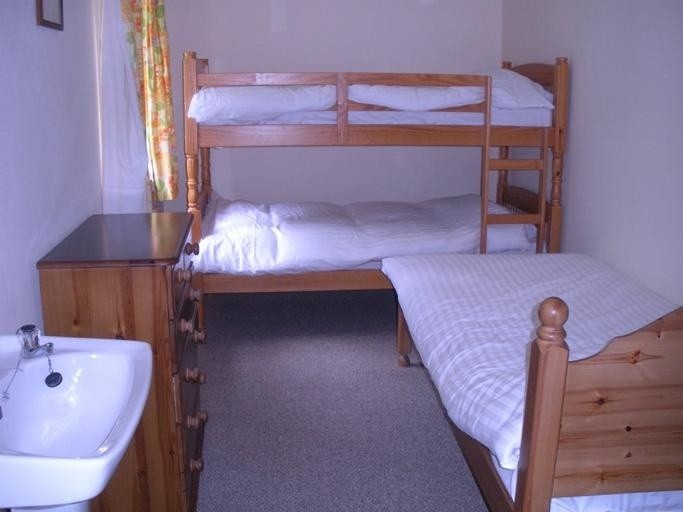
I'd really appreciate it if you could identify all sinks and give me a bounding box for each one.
[0,335,154,510]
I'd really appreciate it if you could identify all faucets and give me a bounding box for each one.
[17,324,54,359]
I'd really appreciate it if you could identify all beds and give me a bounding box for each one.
[181,52,569,331]
[398,252,682,512]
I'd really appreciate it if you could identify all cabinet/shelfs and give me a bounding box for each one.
[35,212,210,512]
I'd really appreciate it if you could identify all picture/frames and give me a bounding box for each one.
[36,0,63,30]
